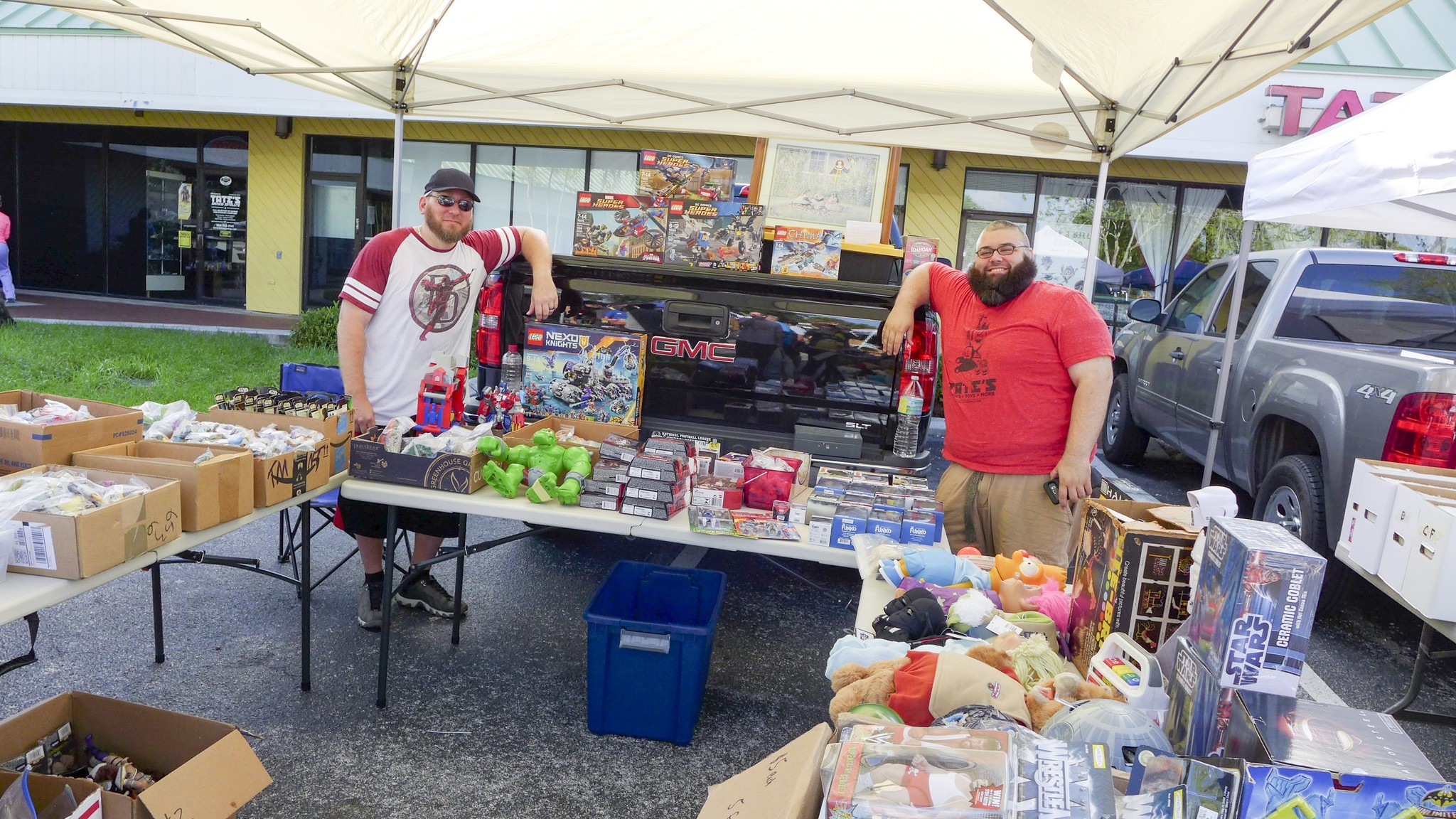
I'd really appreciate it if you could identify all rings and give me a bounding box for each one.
[549,308,555,310]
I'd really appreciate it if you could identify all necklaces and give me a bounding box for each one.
[418,225,425,238]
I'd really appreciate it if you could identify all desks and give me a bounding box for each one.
[1333,542,1456,730]
[0,468,353,692]
[341,477,955,716]
[849,567,1073,648]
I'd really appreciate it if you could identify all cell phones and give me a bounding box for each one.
[1043,477,1070,505]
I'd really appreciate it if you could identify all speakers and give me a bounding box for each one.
[276,116,293,138]
[933,150,947,169]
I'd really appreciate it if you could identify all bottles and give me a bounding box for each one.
[893,376,925,458]
[500,344,522,393]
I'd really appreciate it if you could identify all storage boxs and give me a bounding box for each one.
[0,319,1456,819]
[571,148,844,281]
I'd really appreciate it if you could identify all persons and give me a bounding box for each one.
[882,220,1117,569]
[334,168,559,629]
[0,196,17,304]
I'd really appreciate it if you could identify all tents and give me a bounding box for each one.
[1026,225,1209,304]
[1198,68,1456,492]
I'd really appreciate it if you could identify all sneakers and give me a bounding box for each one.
[396,564,468,618]
[357,581,391,629]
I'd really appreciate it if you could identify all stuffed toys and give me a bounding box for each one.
[822,547,1127,739]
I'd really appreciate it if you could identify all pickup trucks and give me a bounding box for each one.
[1102,245,1455,620]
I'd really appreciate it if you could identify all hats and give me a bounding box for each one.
[424,168,481,204]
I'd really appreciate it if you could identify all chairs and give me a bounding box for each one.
[279,361,414,598]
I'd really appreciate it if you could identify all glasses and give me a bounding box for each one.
[427,193,474,211]
[976,245,1029,259]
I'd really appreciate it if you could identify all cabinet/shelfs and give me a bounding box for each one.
[146,170,186,297]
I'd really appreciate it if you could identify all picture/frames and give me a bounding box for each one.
[746,138,903,244]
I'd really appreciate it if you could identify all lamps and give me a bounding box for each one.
[930,150,947,172]
[274,116,293,139]
